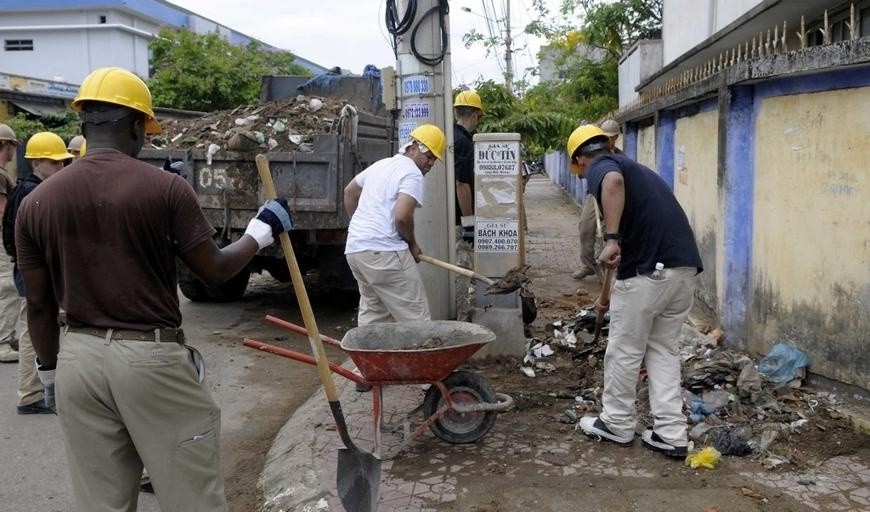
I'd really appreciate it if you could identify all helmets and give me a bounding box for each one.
[66,135,88,158]
[598,119,622,138]
[23,129,78,162]
[407,123,449,165]
[67,65,168,140]
[451,89,485,115]
[564,123,612,174]
[0,123,22,148]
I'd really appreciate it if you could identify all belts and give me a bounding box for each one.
[58,322,189,347]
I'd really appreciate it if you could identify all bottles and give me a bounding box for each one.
[649,262,666,281]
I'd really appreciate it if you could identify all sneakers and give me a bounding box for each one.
[0,340,20,366]
[419,383,434,393]
[352,379,376,393]
[577,415,637,446]
[570,265,596,280]
[640,429,691,460]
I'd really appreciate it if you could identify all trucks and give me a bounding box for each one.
[134,75,398,309]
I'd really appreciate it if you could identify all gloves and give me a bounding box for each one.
[241,195,298,253]
[36,365,59,417]
[459,213,477,248]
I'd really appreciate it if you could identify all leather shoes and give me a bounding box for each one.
[13,395,61,416]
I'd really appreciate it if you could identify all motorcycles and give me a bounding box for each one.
[522,153,546,176]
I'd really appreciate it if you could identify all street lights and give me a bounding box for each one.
[459,1,512,133]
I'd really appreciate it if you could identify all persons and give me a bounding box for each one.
[453,89,486,321]
[0,124,88,413]
[343,125,432,392]
[566,124,703,459]
[140,467,155,494]
[571,118,628,279]
[13,68,293,512]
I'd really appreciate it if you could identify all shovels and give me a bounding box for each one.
[417,254,529,296]
[255,153,382,512]
[572,253,619,359]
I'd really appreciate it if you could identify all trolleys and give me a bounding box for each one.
[243,315,516,460]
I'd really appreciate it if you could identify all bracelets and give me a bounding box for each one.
[603,234,619,242]
